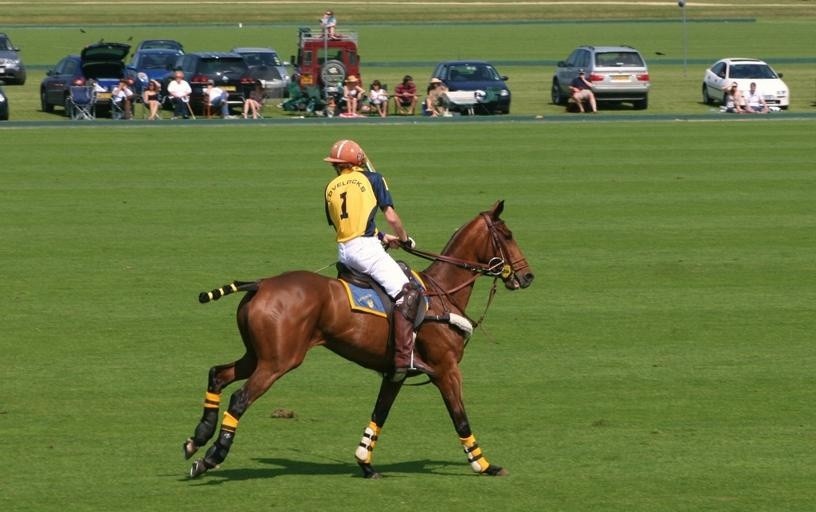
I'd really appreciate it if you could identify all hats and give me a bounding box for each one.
[344,75,357,82]
[429,77,441,83]
[578,67,585,74]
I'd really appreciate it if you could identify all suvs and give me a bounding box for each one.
[548,43,652,108]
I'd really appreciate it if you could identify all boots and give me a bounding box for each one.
[391,304,437,375]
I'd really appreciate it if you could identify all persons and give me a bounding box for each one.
[113,78,134,119]
[322,137,438,374]
[144,79,162,118]
[204,80,230,118]
[318,7,340,40]
[743,81,772,116]
[370,80,389,118]
[168,71,192,119]
[722,79,744,115]
[241,79,261,118]
[568,68,600,114]
[394,75,417,114]
[427,78,450,116]
[342,75,365,115]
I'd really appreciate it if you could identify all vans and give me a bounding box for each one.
[294,29,363,95]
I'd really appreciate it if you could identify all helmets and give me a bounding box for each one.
[322,139,366,165]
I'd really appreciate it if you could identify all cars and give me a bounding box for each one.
[38,38,289,125]
[432,61,513,116]
[0,33,28,84]
[1,90,13,120]
[701,53,790,107]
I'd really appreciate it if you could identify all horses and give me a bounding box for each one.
[183,199,534,480]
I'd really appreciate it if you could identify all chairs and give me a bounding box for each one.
[473,86,501,115]
[202,87,230,119]
[67,84,95,121]
[335,83,389,115]
[109,83,167,120]
